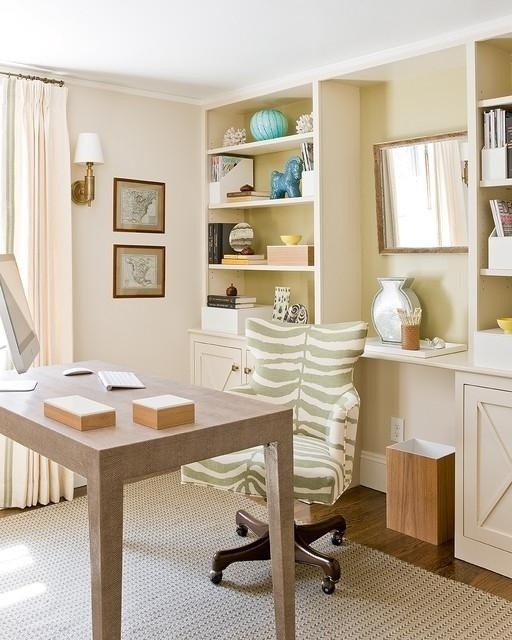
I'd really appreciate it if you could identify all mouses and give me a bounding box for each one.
[63,368,92,376]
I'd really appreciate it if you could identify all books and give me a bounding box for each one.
[221,258,266,265]
[209,294,257,304]
[226,191,270,197]
[483,109,512,237]
[225,196,269,202]
[207,300,256,309]
[211,155,246,182]
[223,253,265,259]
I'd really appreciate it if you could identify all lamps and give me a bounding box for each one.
[71,131,106,208]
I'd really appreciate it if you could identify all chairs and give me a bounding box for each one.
[180,315,369,596]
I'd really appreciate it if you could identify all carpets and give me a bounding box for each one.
[0,469,512,640]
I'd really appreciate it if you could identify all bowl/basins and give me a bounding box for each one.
[280,235,302,245]
[495,317,512,333]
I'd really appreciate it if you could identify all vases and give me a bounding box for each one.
[369,276,423,344]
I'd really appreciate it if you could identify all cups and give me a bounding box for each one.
[400,323,420,351]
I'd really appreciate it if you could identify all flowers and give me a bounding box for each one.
[221,126,247,148]
[294,111,314,134]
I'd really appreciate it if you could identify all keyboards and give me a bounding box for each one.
[98,370,146,390]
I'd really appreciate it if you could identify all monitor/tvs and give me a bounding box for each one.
[0,253,39,392]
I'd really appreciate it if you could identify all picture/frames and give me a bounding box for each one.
[112,176,167,299]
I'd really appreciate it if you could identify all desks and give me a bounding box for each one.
[1,357,297,640]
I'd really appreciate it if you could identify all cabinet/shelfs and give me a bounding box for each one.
[465,31,512,352]
[452,371,512,579]
[186,327,253,392]
[200,79,362,325]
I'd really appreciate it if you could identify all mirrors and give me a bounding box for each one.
[371,130,468,256]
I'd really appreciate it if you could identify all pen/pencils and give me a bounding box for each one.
[396,306,423,327]
